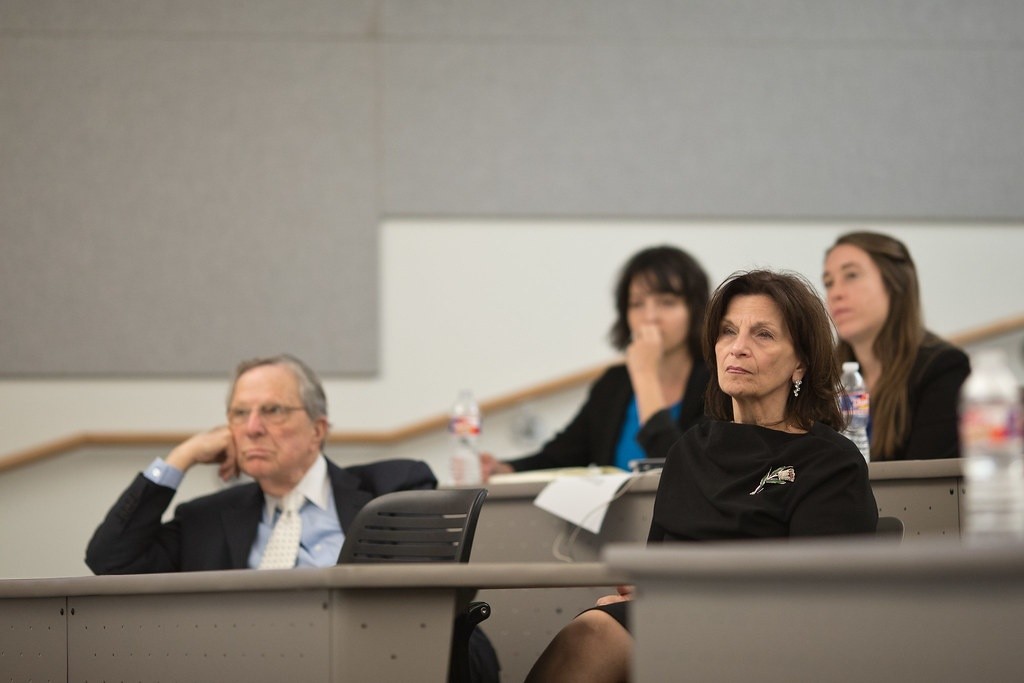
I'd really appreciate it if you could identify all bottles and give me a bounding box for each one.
[448,388,483,487]
[959,350,1024,535]
[835,362,870,462]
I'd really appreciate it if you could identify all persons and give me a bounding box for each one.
[453,246,712,487]
[523,270,878,683]
[823,232,972,462]
[85,354,502,683]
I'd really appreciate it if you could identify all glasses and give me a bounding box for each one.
[227,404,309,427]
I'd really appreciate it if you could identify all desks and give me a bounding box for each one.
[0,458,1024,683]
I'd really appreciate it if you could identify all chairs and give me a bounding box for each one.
[335,489,491,683]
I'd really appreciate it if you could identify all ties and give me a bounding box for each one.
[259,496,311,570]
